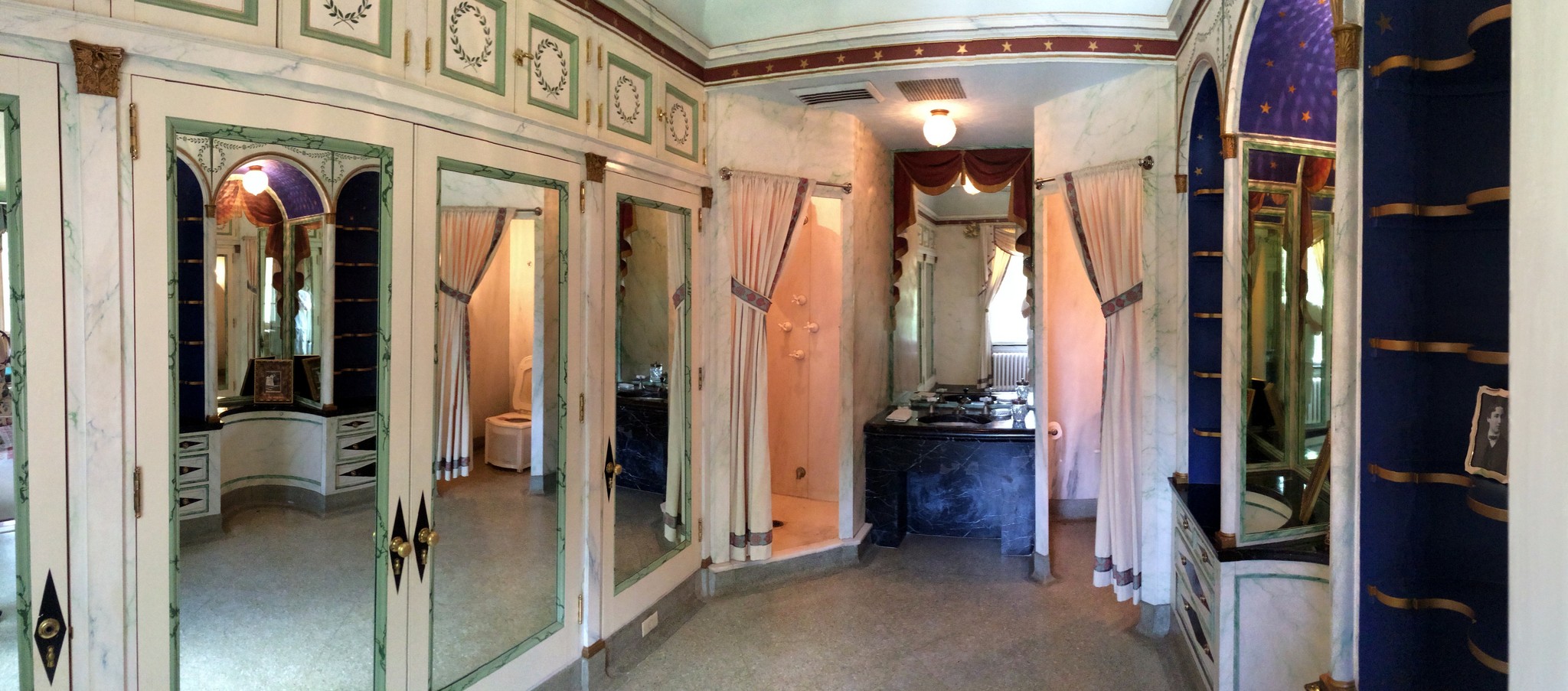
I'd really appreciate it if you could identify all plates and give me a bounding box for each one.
[990,411,1013,417]
[997,401,1012,404]
[618,386,634,389]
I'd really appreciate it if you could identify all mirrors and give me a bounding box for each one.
[1297,167,1337,493]
[214,178,285,403]
[1240,140,1331,544]
[290,220,323,407]
[612,191,692,598]
[1248,186,1294,469]
[887,174,1036,410]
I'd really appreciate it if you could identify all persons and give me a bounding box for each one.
[1283,269,1323,408]
[1470,402,1509,476]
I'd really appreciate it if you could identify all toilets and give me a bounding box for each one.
[483,355,534,473]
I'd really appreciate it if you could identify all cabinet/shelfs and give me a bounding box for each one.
[597,0,709,177]
[1365,4,1511,675]
[1169,490,1331,691]
[515,0,658,158]
[1191,187,1225,438]
[17,0,277,47]
[276,0,516,113]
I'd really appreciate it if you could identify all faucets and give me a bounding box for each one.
[954,396,972,416]
[664,372,667,383]
[656,377,666,392]
[958,388,972,404]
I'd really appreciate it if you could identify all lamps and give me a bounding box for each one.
[923,109,957,147]
[962,174,980,195]
[242,165,269,196]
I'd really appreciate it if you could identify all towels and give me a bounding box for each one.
[885,408,913,422]
[919,392,936,400]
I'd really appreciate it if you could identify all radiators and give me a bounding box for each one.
[984,351,1033,392]
[1305,376,1322,425]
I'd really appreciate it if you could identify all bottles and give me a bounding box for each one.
[650,361,662,382]
[1012,396,1028,422]
[1016,378,1030,400]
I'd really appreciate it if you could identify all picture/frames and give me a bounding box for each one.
[1299,426,1331,526]
[1262,381,1285,439]
[1463,384,1509,484]
[254,359,293,404]
[301,356,321,401]
[239,355,276,397]
[293,355,320,393]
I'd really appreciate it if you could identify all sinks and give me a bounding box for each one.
[642,390,668,400]
[942,393,998,402]
[616,389,655,398]
[917,413,994,426]
[1243,481,1294,535]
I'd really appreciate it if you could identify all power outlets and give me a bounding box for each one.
[641,611,658,638]
